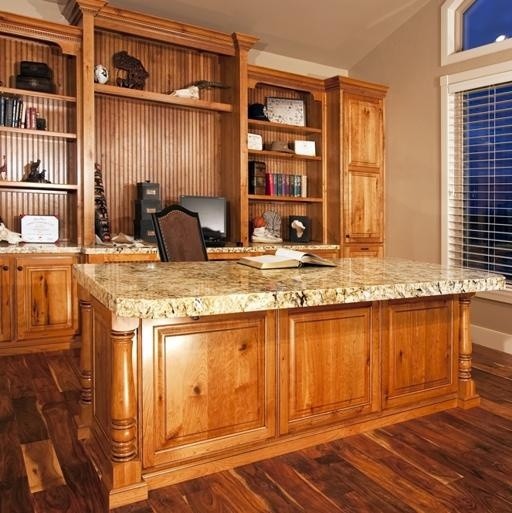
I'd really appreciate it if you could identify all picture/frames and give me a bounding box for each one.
[264,95,308,126]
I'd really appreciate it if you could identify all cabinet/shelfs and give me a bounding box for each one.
[247,65,390,258]
[57,1,262,257]
[0,8,83,358]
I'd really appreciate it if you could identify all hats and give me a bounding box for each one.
[266,140,294,153]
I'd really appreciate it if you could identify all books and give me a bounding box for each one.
[237,246,338,272]
[249,160,307,198]
[0,93,46,130]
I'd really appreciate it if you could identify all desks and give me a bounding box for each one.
[69,256,509,507]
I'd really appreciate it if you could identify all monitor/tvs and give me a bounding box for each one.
[180,195,226,247]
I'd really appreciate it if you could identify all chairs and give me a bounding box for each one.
[150,202,209,262]
